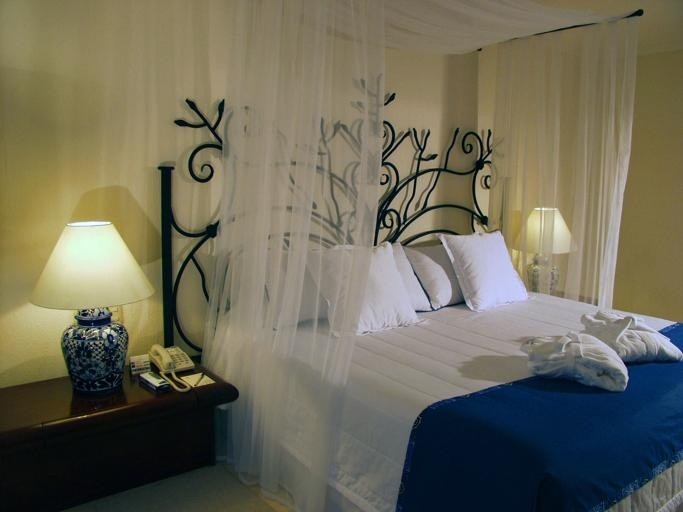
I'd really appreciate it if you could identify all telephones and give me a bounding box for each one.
[148,344,196,392]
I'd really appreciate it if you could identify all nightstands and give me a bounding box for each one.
[550,290,599,304]
[0,355,240,512]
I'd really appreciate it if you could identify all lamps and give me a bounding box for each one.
[29,220,157,395]
[512,207,578,295]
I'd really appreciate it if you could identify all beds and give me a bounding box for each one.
[160,72,683,512]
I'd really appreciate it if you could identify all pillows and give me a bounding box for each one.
[250,244,433,331]
[404,243,461,309]
[304,242,428,340]
[436,230,529,311]
